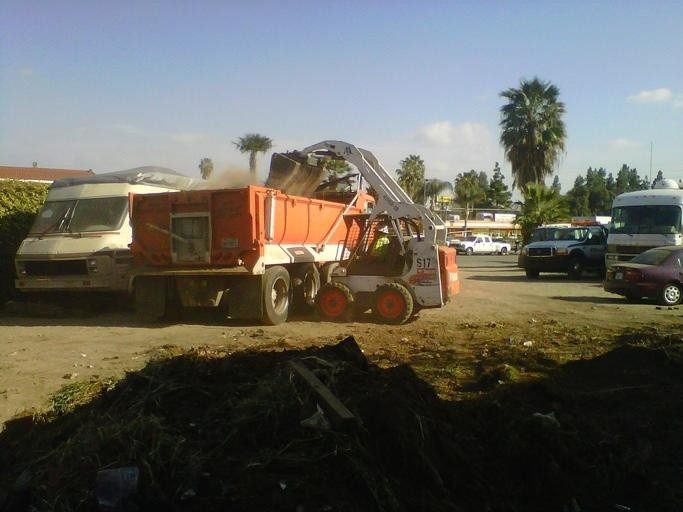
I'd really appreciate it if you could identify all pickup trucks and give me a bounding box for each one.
[448,234,511,255]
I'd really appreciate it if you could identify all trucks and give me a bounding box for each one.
[605,178,683,269]
[15,165,181,303]
[129,185,374,324]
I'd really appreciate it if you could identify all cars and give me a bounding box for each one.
[518,225,683,306]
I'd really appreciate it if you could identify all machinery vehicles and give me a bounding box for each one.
[264,140,461,324]
